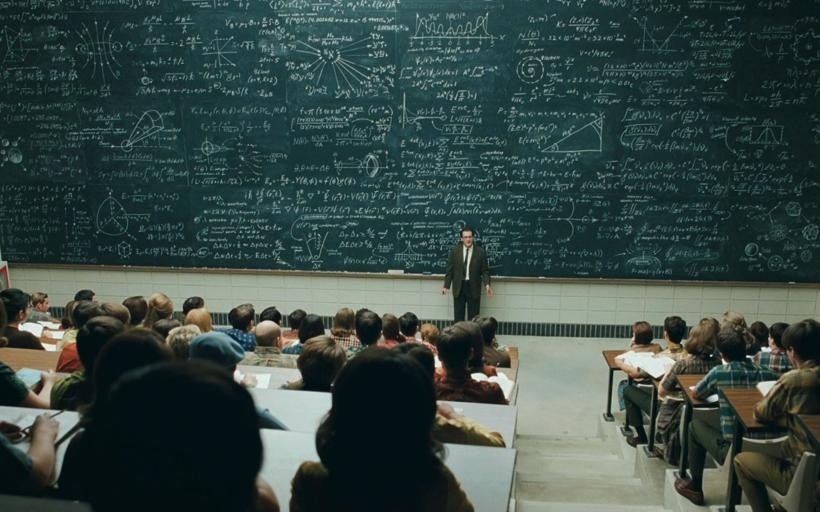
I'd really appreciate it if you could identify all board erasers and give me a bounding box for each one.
[387,270,405,274]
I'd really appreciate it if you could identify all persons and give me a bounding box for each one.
[442,227,492,323]
[619,312,818,512]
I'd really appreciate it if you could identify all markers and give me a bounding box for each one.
[422,271,432,274]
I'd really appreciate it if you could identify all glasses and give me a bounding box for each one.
[462,235,473,240]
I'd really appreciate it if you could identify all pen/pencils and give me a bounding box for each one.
[28,409,65,430]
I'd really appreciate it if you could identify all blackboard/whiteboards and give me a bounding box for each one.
[0,0,820,288]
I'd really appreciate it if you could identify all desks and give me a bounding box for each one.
[601,346,819,511]
[0,325,519,512]
